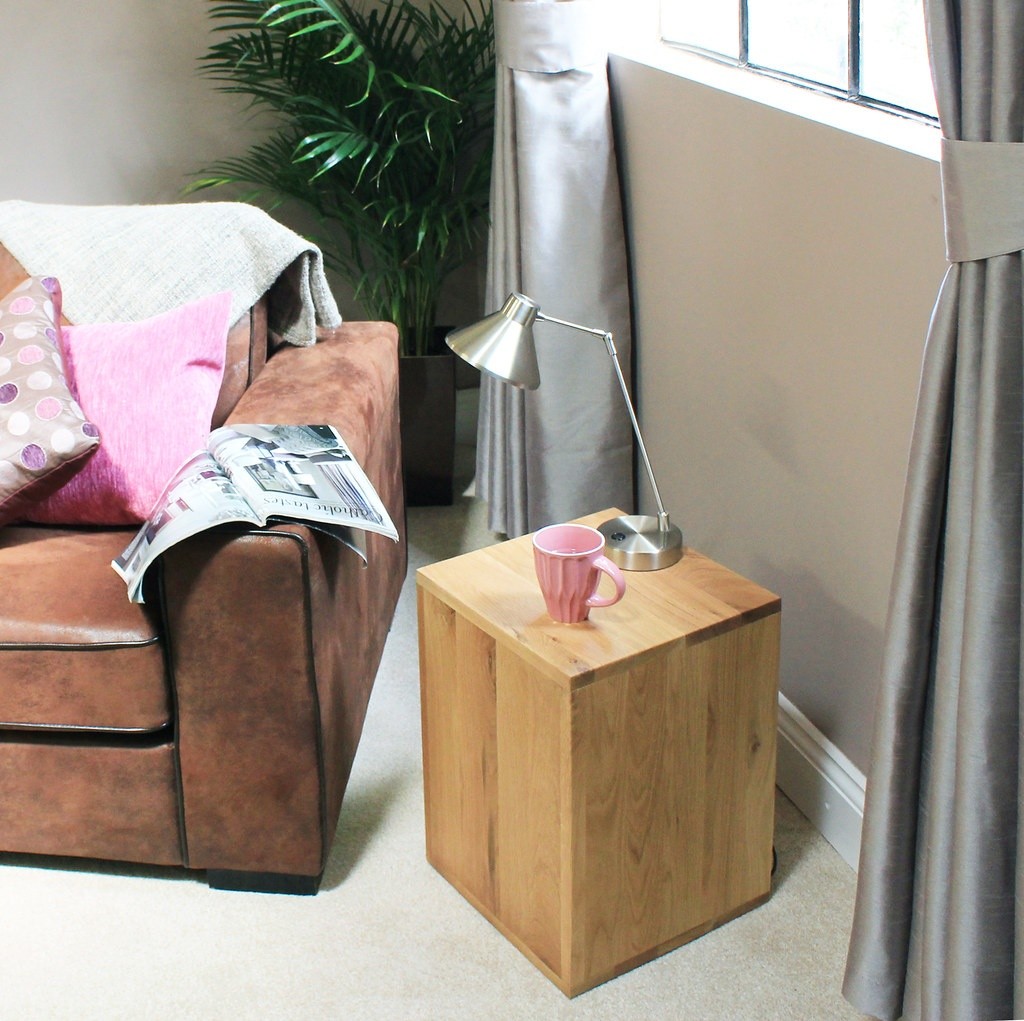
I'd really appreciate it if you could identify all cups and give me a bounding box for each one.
[533,523,626,623]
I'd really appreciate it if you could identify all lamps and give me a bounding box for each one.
[444,292,684,571]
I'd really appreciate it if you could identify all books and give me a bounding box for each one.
[112,423,399,605]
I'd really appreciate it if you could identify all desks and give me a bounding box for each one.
[415,507,782,998]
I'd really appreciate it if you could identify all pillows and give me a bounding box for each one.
[0,273,98,511]
[29,290,233,528]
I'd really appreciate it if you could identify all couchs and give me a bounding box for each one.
[0,197,409,896]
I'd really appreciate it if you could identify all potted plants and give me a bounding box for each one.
[176,0,495,507]
